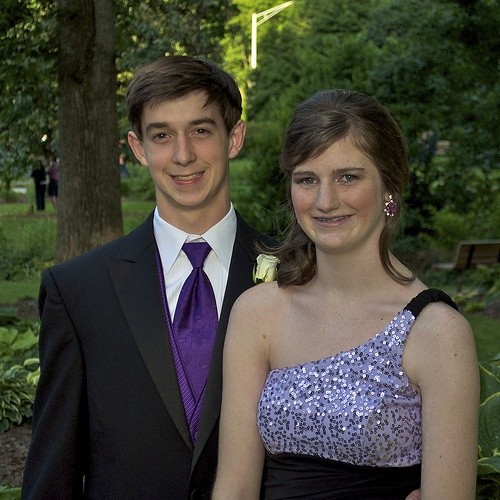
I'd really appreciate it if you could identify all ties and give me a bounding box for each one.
[173,241,220,403]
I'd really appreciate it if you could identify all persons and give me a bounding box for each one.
[21,56,422,500]
[32,155,59,211]
[209,89,479,500]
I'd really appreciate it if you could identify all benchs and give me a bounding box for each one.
[431,240,500,292]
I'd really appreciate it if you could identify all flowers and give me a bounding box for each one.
[253,254,280,285]
